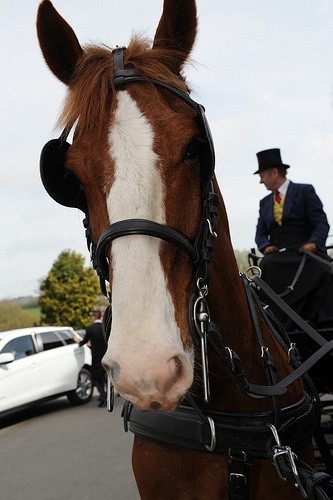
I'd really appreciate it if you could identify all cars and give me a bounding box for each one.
[75,329,92,347]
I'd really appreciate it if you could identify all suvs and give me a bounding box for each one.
[0,326,94,419]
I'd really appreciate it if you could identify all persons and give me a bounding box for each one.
[73,310,108,408]
[255,160,330,256]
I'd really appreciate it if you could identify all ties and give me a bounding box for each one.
[274,191,281,204]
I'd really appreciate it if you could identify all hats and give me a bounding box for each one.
[253,148,290,174]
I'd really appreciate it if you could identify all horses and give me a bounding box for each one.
[35,0,316,500]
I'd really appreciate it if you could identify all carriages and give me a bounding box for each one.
[37,0,333,500]
